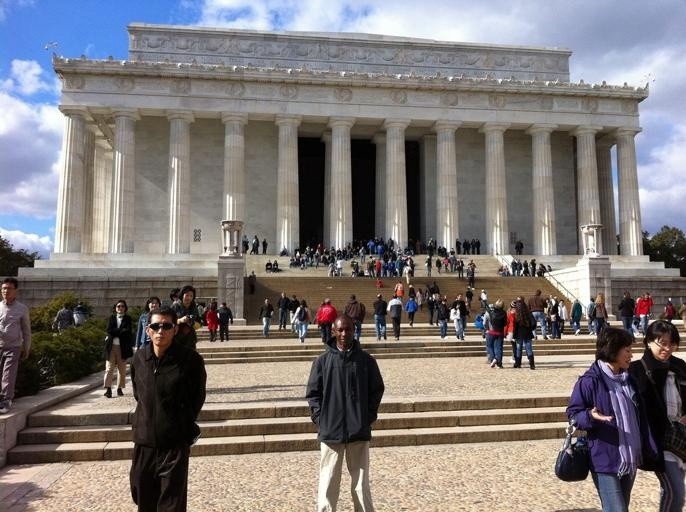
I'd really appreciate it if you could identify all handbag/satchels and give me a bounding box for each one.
[663,416,685,460]
[554,410,591,482]
[646,312,654,320]
[449,307,461,321]
[602,317,610,328]
[358,301,365,323]
[528,310,537,331]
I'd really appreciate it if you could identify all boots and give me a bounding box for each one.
[513,357,522,368]
[527,353,536,369]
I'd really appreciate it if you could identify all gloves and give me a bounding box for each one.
[179,315,189,324]
[231,320,233,324]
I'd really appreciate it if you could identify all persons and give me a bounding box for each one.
[52,302,90,333]
[129,306,207,512]
[0,277,31,415]
[497,239,552,276]
[304,313,386,512]
[242,233,481,283]
[103,286,233,398]
[482,295,535,370]
[373,282,686,340]
[625,319,686,512]
[259,292,366,341]
[566,326,660,512]
[248,270,256,292]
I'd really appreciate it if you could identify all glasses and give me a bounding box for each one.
[147,322,176,330]
[116,305,127,309]
[653,339,678,352]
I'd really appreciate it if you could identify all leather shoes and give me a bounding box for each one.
[103,386,113,398]
[116,387,124,396]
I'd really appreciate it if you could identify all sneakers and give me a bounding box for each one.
[208,337,230,342]
[0,397,13,414]
[376,335,466,341]
[542,329,598,340]
[407,318,439,328]
[490,358,504,369]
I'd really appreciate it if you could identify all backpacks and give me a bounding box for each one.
[298,306,308,321]
[488,307,508,332]
